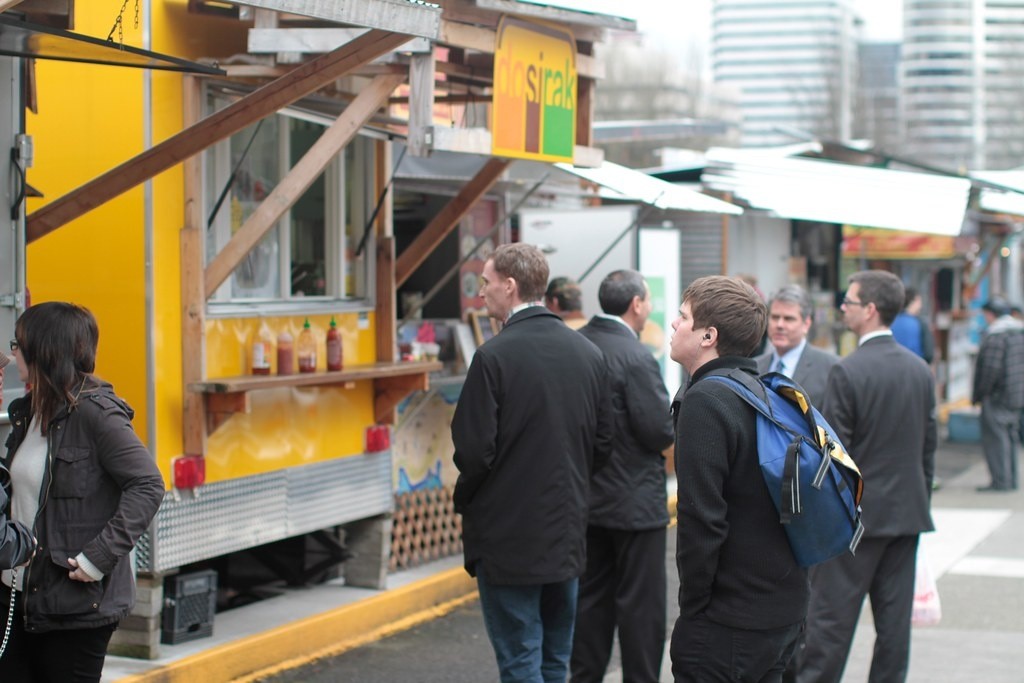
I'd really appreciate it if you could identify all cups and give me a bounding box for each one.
[400,290,423,319]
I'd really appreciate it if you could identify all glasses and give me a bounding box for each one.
[843,297,866,306]
[10,339,19,351]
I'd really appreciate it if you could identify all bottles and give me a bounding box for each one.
[326,316,342,371]
[278,325,294,376]
[252,319,272,376]
[297,319,317,374]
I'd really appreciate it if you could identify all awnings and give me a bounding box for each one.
[185,0,639,164]
[820,140,1024,301]
[392,161,745,217]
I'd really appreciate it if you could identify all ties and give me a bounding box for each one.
[774,360,784,375]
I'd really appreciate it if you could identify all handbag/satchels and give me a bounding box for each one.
[911,533,941,627]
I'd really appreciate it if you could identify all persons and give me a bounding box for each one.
[0,301,166,683]
[971,297,1024,491]
[750,286,847,413]
[668,274,812,683]
[889,288,935,365]
[568,269,675,683]
[796,270,938,683]
[451,243,617,683]
[0,351,38,571]
[545,277,589,331]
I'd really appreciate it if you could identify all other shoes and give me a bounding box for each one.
[975,481,1018,490]
[930,478,940,491]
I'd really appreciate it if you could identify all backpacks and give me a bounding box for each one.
[699,372,865,570]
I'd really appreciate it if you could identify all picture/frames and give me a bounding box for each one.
[469,311,499,349]
